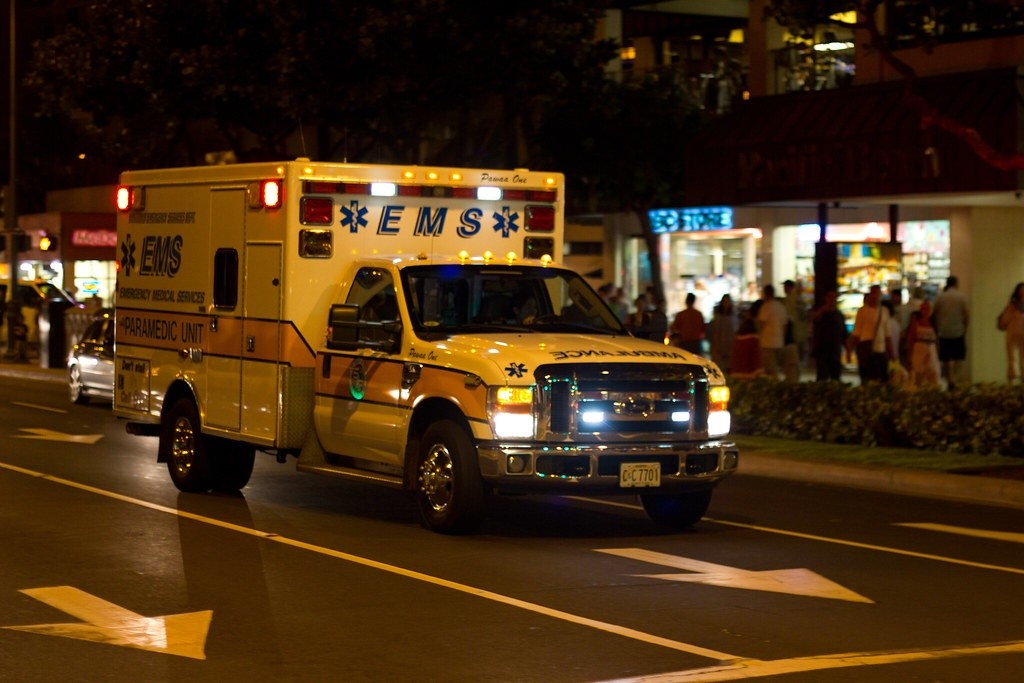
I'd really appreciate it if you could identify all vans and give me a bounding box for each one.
[0,279,75,343]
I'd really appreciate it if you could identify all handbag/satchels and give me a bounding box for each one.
[887,360,909,389]
[855,340,873,361]
[997,312,1009,330]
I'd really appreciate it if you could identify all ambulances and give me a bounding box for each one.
[116,157,741,535]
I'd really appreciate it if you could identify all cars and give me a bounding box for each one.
[62,316,115,404]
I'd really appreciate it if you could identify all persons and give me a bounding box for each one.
[755,285,788,376]
[850,280,944,390]
[671,293,704,355]
[709,296,740,373]
[807,290,850,382]
[599,284,629,326]
[641,286,668,343]
[997,282,1024,384]
[929,276,970,390]
[779,279,802,383]
[631,295,652,339]
[508,291,542,324]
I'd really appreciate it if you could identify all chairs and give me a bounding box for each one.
[478,295,512,324]
